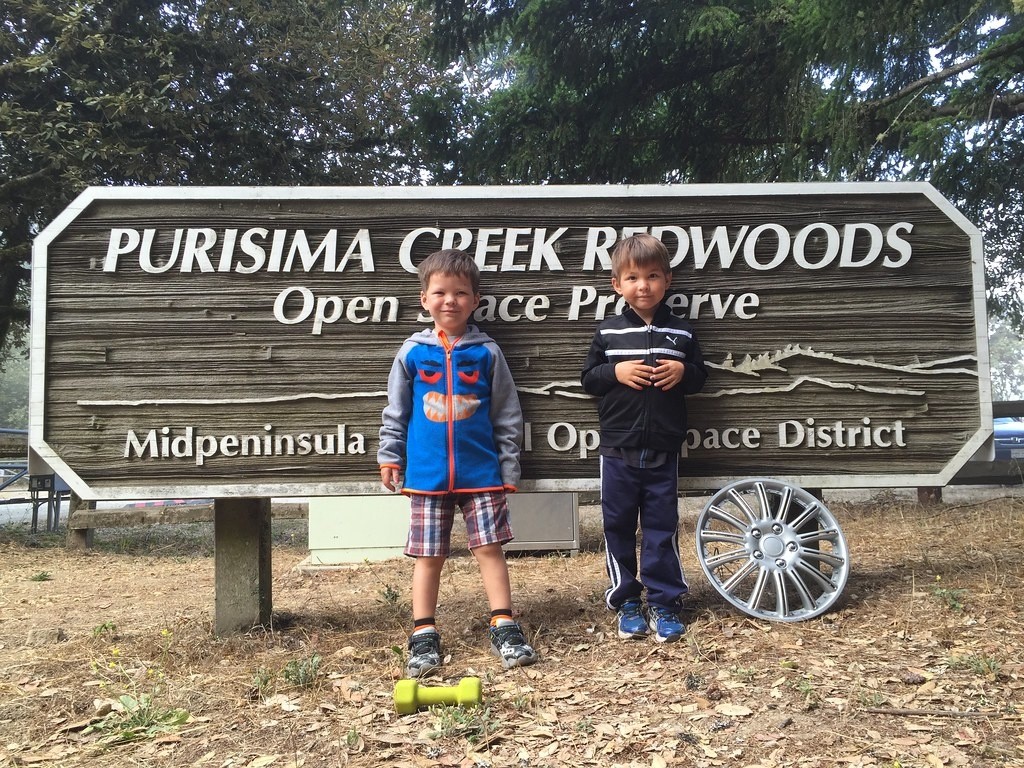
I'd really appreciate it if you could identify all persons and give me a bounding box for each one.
[377,248,536,678]
[580,232,708,644]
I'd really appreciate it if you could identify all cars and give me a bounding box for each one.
[950,416,1023,484]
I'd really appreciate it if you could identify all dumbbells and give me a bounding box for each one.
[394,676,483,715]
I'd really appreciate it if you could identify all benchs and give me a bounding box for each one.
[0,429,69,532]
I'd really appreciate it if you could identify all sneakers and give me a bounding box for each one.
[647,605,684,643]
[616,600,650,639]
[490,625,538,669]
[407,626,441,677]
